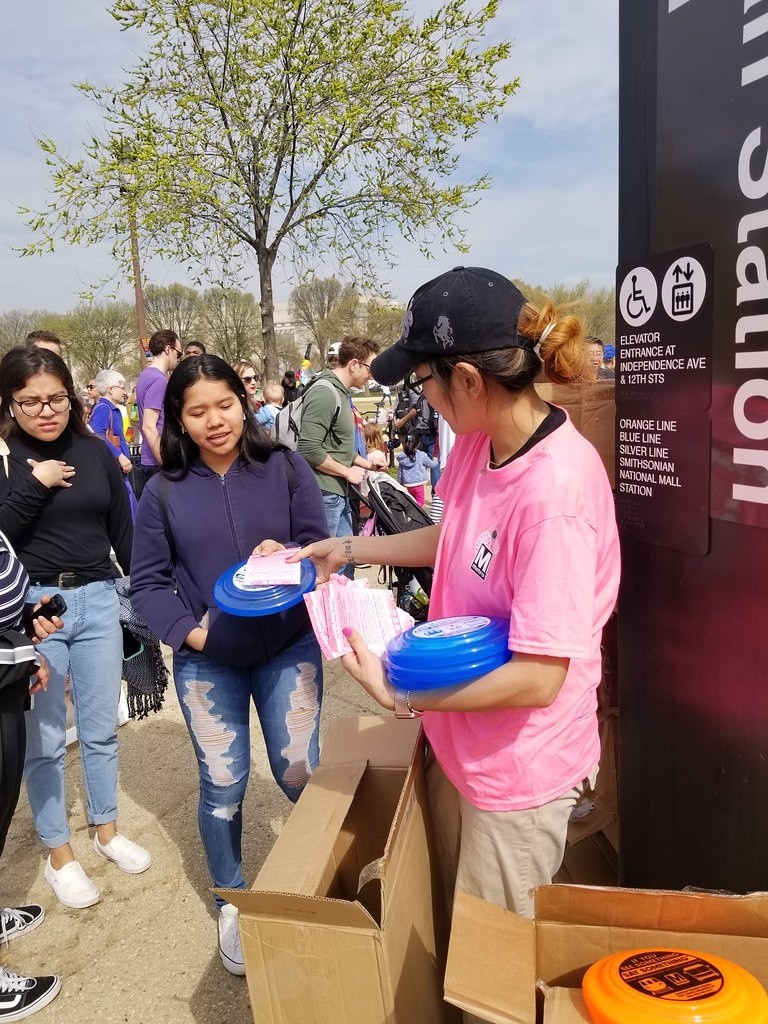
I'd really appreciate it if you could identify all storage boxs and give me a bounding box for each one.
[210,713,440,1023]
[441,883,768,1023]
[535,377,616,490]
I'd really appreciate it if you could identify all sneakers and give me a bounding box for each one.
[0,966,61,1021]
[216,903,247,975]
[0,904,45,944]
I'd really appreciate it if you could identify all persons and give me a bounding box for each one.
[130,352,328,974]
[0,345,152,909]
[298,334,455,582]
[83,369,142,527]
[0,532,64,1024]
[185,341,206,358]
[137,329,183,494]
[284,266,622,921]
[231,362,298,437]
[582,337,615,379]
[26,331,60,356]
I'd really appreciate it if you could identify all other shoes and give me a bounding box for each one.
[94,832,151,873]
[45,854,99,908]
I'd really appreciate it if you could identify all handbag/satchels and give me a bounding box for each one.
[89,403,120,450]
[360,511,377,536]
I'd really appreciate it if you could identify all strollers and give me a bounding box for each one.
[348,465,438,624]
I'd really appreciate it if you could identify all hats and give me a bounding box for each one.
[603,344,615,359]
[285,371,297,381]
[370,266,535,386]
[328,342,342,355]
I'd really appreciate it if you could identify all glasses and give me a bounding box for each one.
[9,394,71,417]
[241,375,260,384]
[404,371,437,395]
[171,346,183,358]
[86,385,95,389]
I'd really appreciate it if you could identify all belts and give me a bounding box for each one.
[28,573,94,590]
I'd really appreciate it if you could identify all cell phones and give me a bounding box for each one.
[26,594,68,640]
[123,627,144,661]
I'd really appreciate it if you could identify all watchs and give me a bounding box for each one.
[395,688,422,720]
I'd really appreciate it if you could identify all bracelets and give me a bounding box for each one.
[407,691,424,715]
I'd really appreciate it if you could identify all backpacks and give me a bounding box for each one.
[396,392,411,434]
[270,379,342,452]
